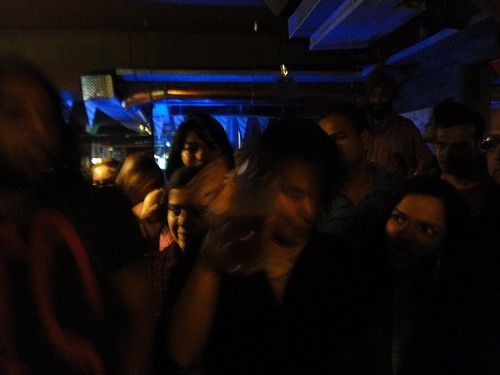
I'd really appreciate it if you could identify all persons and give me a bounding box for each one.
[0,48,500,375]
